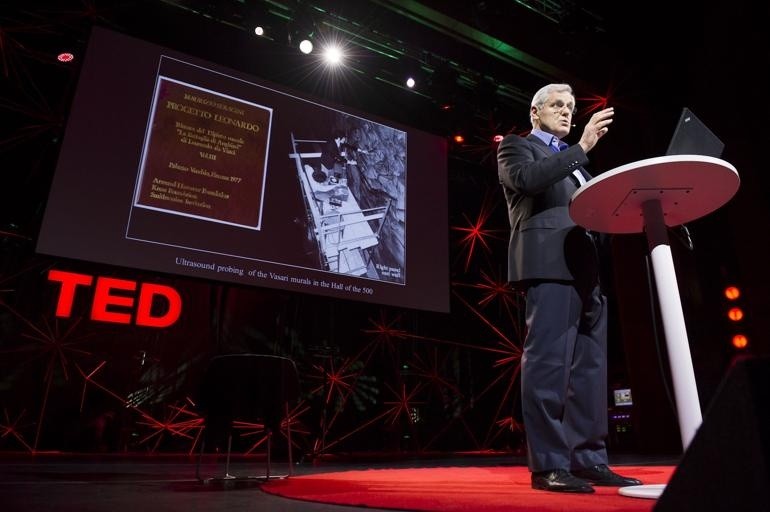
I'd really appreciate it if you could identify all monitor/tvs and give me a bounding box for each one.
[613,387,634,407]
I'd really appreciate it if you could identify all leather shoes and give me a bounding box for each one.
[530,467,594,495]
[572,463,644,488]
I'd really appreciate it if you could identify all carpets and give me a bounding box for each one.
[259,464,678,512]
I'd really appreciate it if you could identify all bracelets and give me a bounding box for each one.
[361,150,362,153]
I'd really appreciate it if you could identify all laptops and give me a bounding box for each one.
[665,107,726,158]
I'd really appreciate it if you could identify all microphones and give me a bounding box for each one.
[571,124,576,127]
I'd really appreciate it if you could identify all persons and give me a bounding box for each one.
[320,130,369,180]
[494,84,645,495]
